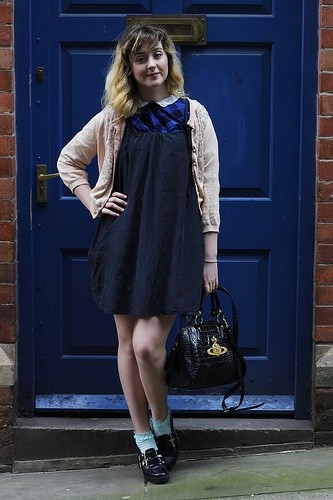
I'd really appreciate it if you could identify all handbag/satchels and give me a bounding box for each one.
[166,284,249,417]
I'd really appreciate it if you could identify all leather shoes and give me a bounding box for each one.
[151,421,180,469]
[138,449,169,485]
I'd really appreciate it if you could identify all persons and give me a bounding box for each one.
[56,18,222,486]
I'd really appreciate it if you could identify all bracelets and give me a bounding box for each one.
[203,258,218,264]
